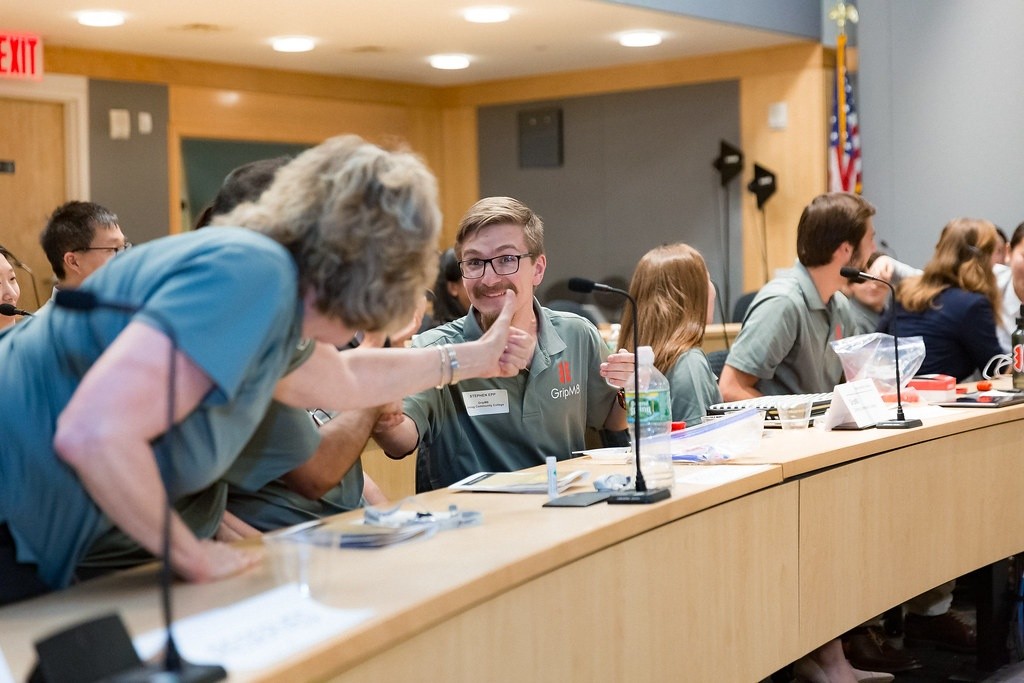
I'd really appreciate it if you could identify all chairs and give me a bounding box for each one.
[706,349,731,385]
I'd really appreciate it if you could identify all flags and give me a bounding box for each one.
[828,34,863,197]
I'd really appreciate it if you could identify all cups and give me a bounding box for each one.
[775,397,813,433]
[700,409,767,447]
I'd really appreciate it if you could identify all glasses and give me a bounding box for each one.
[69,241,132,258]
[458,252,535,279]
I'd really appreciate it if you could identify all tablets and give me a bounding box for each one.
[938,395,1024,407]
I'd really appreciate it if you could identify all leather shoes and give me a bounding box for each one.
[903,611,979,652]
[842,628,921,673]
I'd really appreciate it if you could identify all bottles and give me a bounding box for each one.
[623,345,677,491]
[1012,316,1024,391]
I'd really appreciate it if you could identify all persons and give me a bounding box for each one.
[716,190,980,671]
[994,225,1008,265]
[189,153,385,544]
[838,250,925,336]
[875,217,1020,645]
[990,222,1024,354]
[431,249,472,331]
[0,135,540,610]
[39,199,127,317]
[373,195,639,494]
[612,243,904,682]
[0,245,32,332]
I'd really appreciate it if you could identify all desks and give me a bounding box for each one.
[0,464,800,683]
[561,380,1024,658]
[599,323,742,354]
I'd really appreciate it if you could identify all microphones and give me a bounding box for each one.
[0,303,31,317]
[567,276,672,505]
[840,266,923,429]
[54,290,229,683]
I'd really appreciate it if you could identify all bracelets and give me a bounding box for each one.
[436,345,447,390]
[443,344,461,386]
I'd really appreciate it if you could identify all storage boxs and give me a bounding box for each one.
[907,372,957,405]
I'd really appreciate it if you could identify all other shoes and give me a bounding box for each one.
[792,656,896,683]
